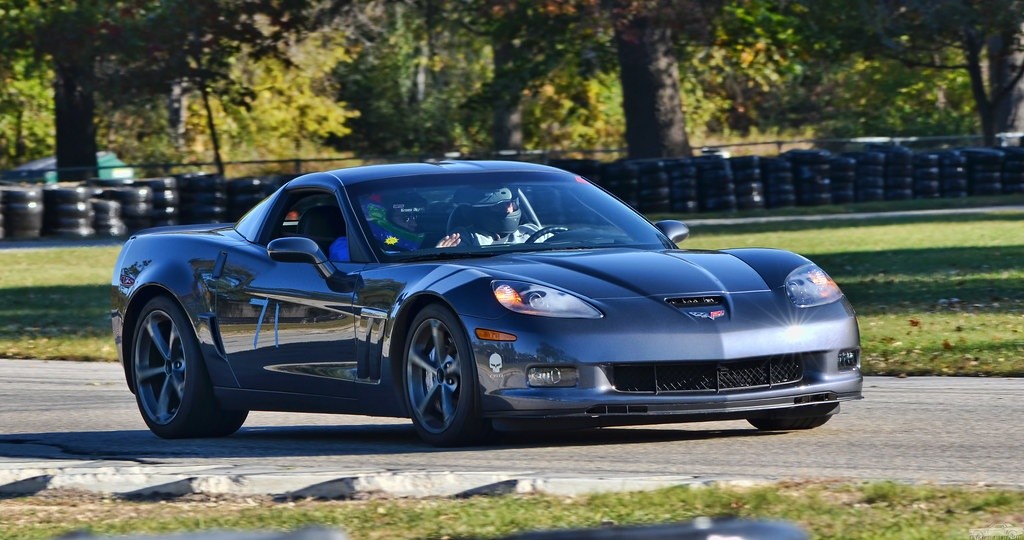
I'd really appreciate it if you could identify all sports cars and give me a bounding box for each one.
[110,158,862,448]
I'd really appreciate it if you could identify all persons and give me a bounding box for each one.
[450,179,557,253]
[327,186,461,265]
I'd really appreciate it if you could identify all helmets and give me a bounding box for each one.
[362,188,427,252]
[469,184,522,233]
